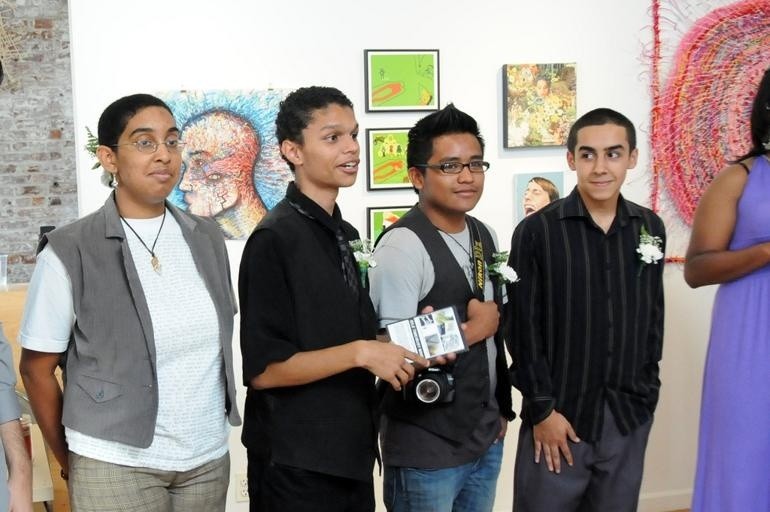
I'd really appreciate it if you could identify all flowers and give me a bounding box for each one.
[488,250,523,288]
[636,222,665,266]
[349,236,378,287]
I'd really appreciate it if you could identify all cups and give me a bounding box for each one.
[0,255,9,288]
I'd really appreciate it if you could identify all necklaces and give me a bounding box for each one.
[115,208,169,273]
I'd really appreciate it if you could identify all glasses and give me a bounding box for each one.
[421,160,490,176]
[116,137,187,154]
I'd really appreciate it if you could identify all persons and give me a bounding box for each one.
[18,91,240,512]
[502,106,667,511]
[525,72,567,146]
[368,101,517,511]
[0,321,37,512]
[522,176,560,218]
[682,68,769,512]
[179,108,269,241]
[236,84,458,511]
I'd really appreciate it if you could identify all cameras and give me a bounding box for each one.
[403,368,454,403]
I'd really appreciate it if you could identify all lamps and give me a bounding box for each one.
[363,48,442,114]
[366,205,416,247]
[365,127,418,192]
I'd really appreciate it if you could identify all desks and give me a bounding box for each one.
[19,423,56,511]
